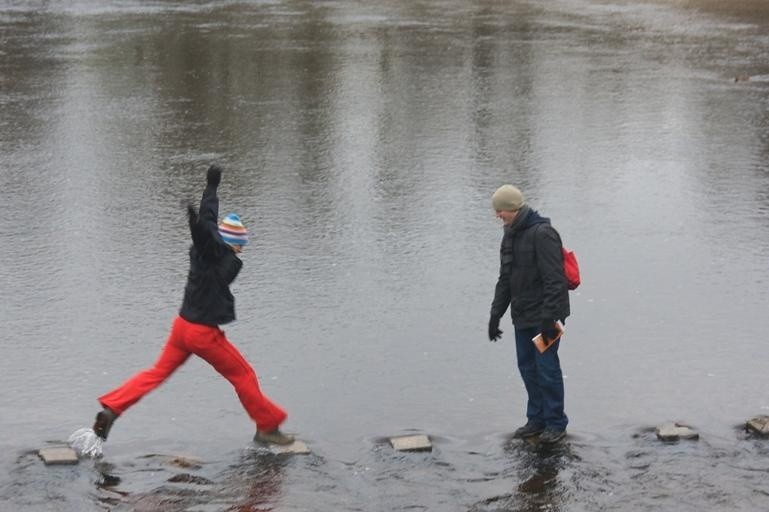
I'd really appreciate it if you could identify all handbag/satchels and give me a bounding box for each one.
[560,246,580,291]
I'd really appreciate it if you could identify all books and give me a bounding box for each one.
[530,319,566,354]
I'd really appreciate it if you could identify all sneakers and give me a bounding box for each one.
[516,416,568,444]
[93,411,112,440]
[252,430,296,447]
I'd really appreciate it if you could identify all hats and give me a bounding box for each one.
[490,184,525,211]
[217,214,249,246]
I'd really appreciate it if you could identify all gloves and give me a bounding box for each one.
[207,164,226,184]
[488,314,503,343]
[540,318,561,347]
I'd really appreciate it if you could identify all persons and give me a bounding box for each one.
[486,185,570,443]
[92,166,295,445]
